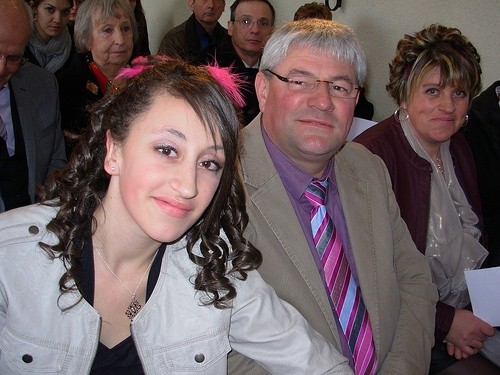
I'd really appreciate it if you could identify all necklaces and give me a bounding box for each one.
[91,242,159,321]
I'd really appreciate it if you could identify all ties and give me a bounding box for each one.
[303,179,378,375]
[0,115,8,144]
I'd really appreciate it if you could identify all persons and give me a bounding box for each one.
[0,55,355,375]
[227,18,438,375]
[449,78,500,270]
[352,23,500,375]
[0,0,374,219]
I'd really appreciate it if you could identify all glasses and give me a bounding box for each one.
[234,18,273,29]
[263,68,360,98]
[0,53,22,63]
